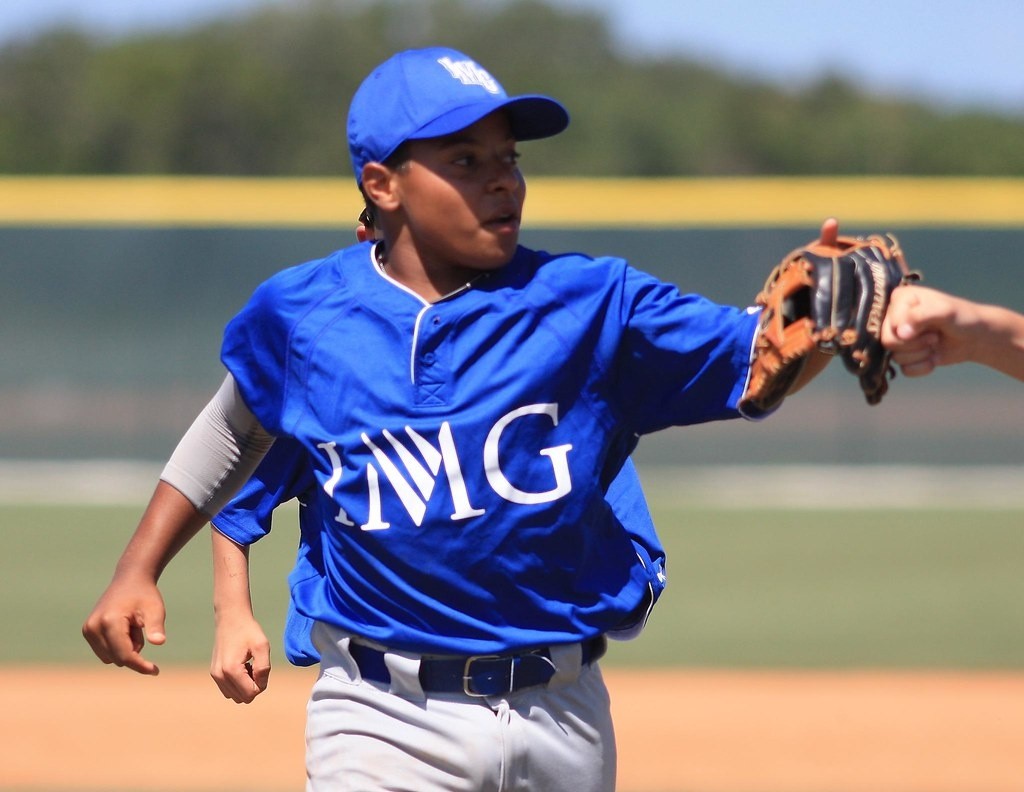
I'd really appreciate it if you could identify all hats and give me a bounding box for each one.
[345,46,570,189]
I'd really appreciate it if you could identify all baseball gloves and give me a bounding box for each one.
[732,230,909,425]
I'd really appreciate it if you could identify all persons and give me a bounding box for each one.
[212,204,667,703]
[81,47,922,792]
[882,285,1024,380]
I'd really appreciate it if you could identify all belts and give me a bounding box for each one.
[350,633,609,697]
